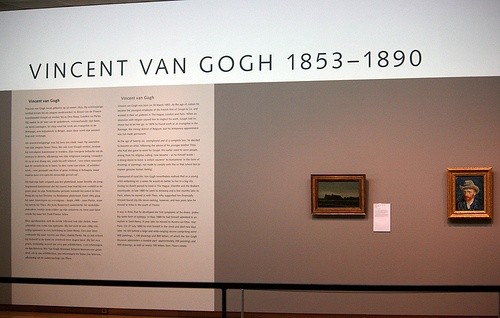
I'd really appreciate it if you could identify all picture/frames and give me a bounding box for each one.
[446,166,494,223]
[309,173,367,218]
